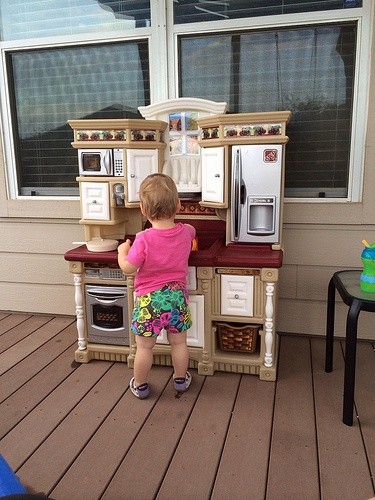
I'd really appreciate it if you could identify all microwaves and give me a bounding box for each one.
[78,148,124,178]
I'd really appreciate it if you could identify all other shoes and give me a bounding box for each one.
[130,377,150,398]
[172,370,192,391]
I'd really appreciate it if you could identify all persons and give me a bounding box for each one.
[117,173,196,399]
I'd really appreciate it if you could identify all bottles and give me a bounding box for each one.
[360,243,375,292]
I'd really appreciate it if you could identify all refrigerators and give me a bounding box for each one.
[231,143,281,243]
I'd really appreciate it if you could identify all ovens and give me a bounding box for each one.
[83,284,129,346]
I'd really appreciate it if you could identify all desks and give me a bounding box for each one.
[325,270,375,426]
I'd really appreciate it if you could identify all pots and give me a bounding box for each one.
[72,239,118,252]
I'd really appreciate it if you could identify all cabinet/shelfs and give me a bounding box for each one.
[63,97,291,383]
